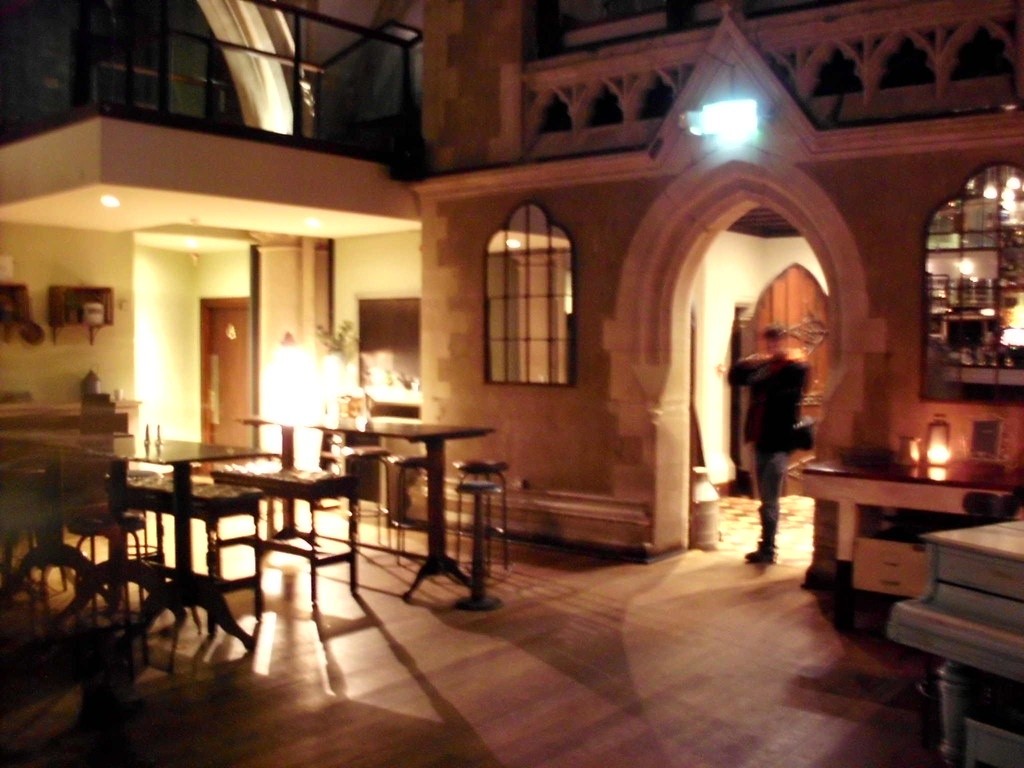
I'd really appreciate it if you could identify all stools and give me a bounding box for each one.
[963,689,1024,768]
[341,444,392,553]
[452,482,504,613]
[451,457,511,578]
[387,454,428,566]
[65,515,150,685]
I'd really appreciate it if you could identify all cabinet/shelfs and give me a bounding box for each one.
[0,281,30,344]
[48,285,115,345]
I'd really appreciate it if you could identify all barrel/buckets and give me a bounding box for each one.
[84,302,104,323]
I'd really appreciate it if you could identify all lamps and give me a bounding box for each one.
[925,413,953,467]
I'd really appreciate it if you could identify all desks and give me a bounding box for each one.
[886,599,1024,768]
[0,409,129,435]
[799,453,1024,599]
[0,428,280,677]
[241,413,495,605]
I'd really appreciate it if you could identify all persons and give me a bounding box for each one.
[727,325,815,564]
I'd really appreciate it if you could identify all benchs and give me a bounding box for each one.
[126,475,264,638]
[208,461,360,613]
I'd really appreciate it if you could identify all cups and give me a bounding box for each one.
[897,438,919,464]
[114,390,122,400]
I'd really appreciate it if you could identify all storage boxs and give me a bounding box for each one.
[916,518,1024,625]
[853,526,928,598]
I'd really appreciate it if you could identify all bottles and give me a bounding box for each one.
[925,415,948,449]
[84,370,100,393]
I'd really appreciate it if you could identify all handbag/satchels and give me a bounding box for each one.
[784,417,816,456]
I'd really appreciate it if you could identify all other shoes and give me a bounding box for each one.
[744,542,776,564]
